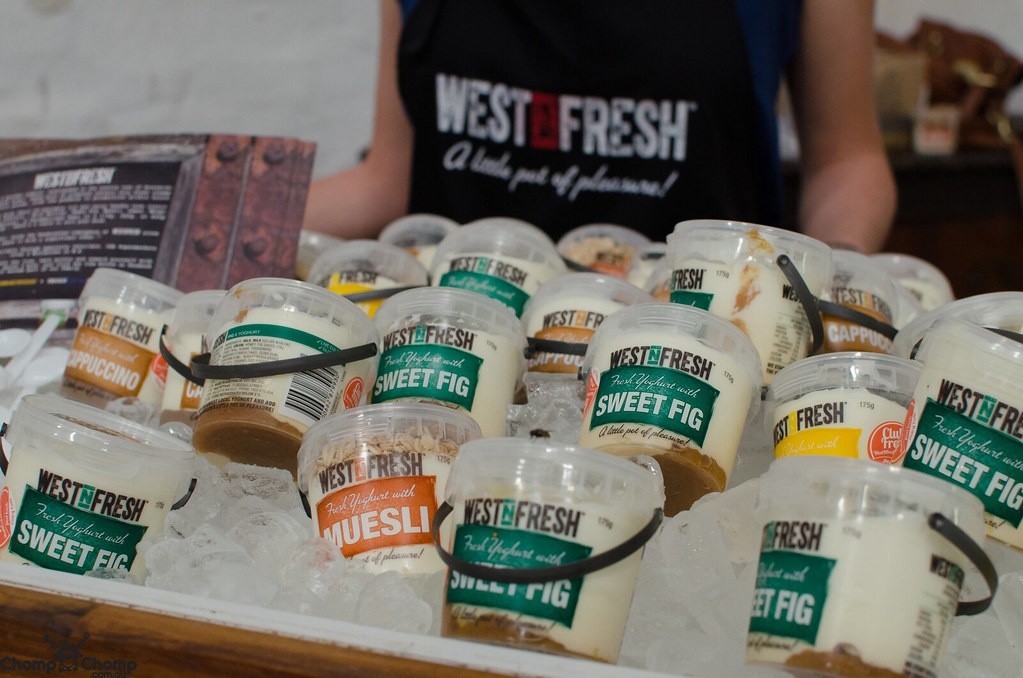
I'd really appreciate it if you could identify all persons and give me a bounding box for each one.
[300,0,894,256]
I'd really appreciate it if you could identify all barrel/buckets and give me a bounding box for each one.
[0,211,1023,678]
